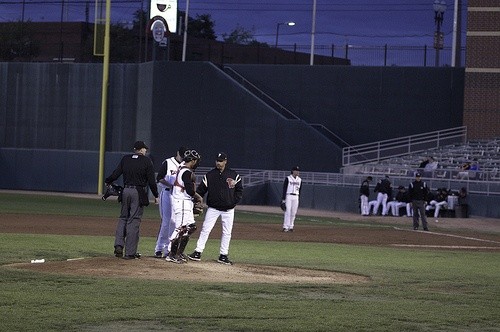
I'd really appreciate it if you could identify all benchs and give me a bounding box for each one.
[371,201,464,218]
[358,140,500,183]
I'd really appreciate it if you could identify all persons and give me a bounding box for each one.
[281,167,302,233]
[166,150,204,265]
[104,141,160,260]
[155,147,187,259]
[359,156,480,231]
[187,152,242,266]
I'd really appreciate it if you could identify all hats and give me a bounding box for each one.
[292,166,302,171]
[431,156,435,160]
[133,141,148,149]
[416,173,421,177]
[215,152,227,160]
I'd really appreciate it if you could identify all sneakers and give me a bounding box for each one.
[165,256,181,263]
[177,253,187,262]
[187,251,202,261]
[217,254,232,265]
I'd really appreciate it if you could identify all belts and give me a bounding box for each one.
[124,185,136,188]
[290,193,296,195]
[165,188,170,191]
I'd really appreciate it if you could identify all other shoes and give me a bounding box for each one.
[114,251,123,257]
[283,228,289,232]
[424,228,430,231]
[124,253,142,259]
[415,227,418,230]
[155,251,167,257]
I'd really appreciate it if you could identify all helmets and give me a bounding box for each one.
[183,150,200,161]
